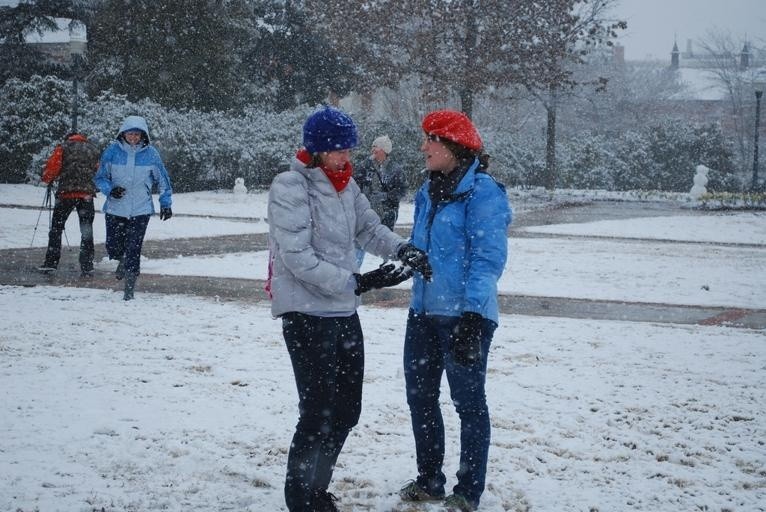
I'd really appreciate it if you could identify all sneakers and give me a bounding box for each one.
[37,264,57,271]
[396,481,445,504]
[116,257,126,280]
[285,488,339,511]
[82,266,95,275]
[123,288,134,300]
[440,492,478,512]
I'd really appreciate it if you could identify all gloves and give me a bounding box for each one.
[160,208,172,221]
[397,243,432,283]
[446,310,483,372]
[352,263,415,295]
[110,187,125,198]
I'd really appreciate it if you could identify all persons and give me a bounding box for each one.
[92,115,173,301]
[397,109,513,511]
[353,134,410,272]
[267,107,433,511]
[37,129,101,280]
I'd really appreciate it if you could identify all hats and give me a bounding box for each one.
[421,110,483,151]
[372,134,393,155]
[303,109,356,153]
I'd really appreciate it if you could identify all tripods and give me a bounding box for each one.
[29,187,72,249]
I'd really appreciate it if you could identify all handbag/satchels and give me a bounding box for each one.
[381,184,398,210]
